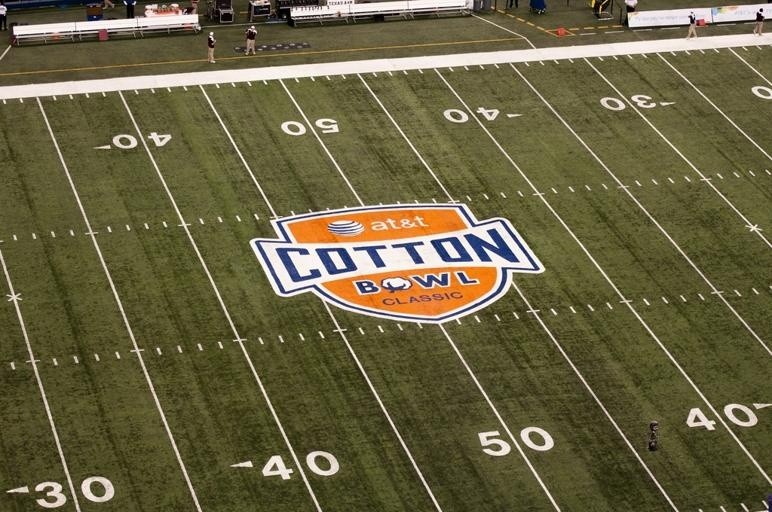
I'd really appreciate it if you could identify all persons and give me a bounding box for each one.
[686,10,698,41]
[180,6,197,16]
[102,1,114,9]
[647,420,659,450]
[244,25,258,56]
[751,7,766,37]
[0,1,9,32]
[623,0,639,26]
[207,31,217,64]
[122,0,137,19]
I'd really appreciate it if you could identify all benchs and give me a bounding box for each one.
[290,0,466,27]
[12,15,202,45]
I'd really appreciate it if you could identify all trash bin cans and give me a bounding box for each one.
[9,22,17,36]
